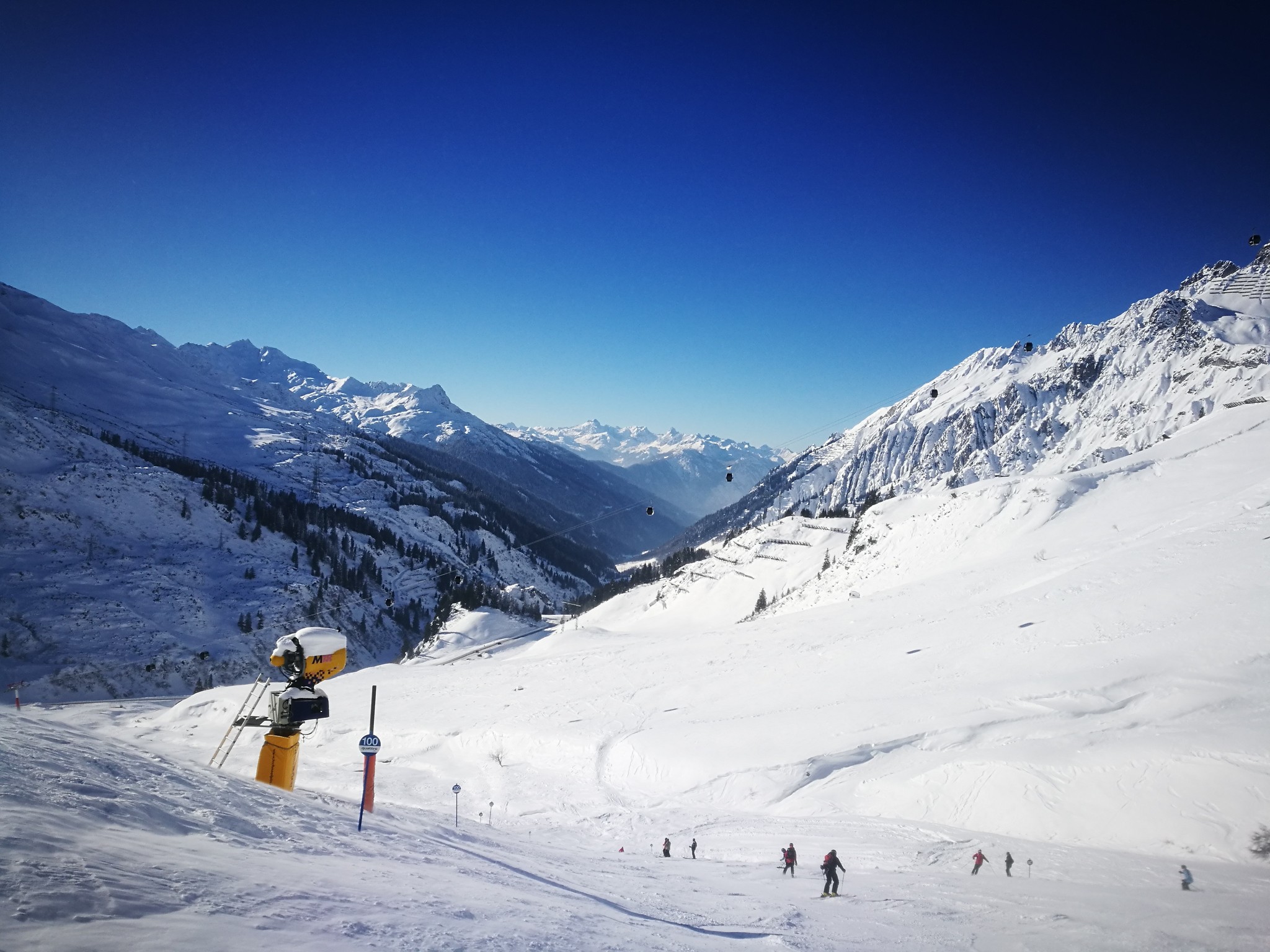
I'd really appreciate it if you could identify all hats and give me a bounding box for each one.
[693,838,695,841]
[789,843,793,846]
[667,839,669,841]
[831,850,837,855]
[978,849,981,852]
[1006,852,1010,855]
[781,848,785,852]
[665,838,667,841]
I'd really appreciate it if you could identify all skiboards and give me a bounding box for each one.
[683,857,691,859]
[810,894,856,900]
[777,866,785,868]
[659,856,663,858]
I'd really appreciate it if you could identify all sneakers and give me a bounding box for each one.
[823,892,831,896]
[831,892,839,896]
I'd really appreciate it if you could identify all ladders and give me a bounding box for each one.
[208,672,272,769]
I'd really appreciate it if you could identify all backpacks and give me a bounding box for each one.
[787,847,795,859]
[824,854,833,866]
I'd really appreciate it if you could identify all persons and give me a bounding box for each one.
[663,838,671,857]
[820,850,846,897]
[1179,865,1193,890]
[780,843,797,878]
[971,849,989,875]
[1005,852,1014,877]
[689,838,697,859]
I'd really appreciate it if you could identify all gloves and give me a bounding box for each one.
[820,865,824,870]
[689,846,691,848]
[796,863,797,866]
[780,859,782,861]
[843,869,846,873]
[663,844,664,845]
